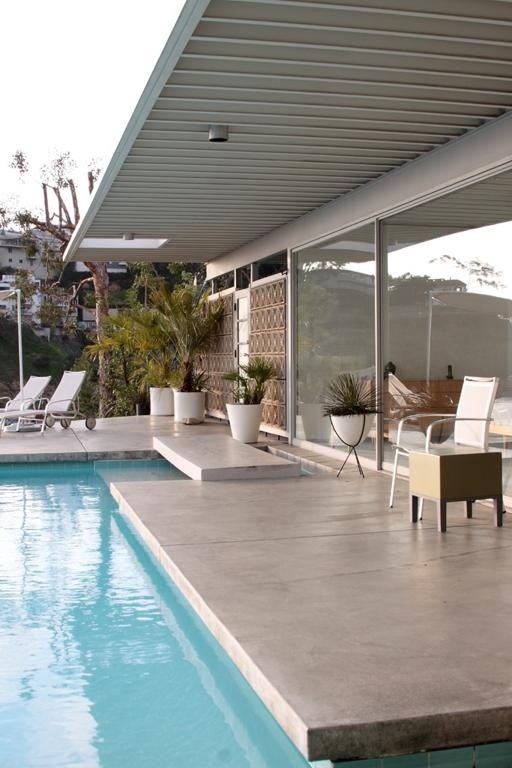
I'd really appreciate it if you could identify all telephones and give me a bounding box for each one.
[446,365,453,379]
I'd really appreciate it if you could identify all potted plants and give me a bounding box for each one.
[86,281,231,425]
[320,369,383,445]
[221,356,279,445]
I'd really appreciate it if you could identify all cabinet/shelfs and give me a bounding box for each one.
[379,380,470,439]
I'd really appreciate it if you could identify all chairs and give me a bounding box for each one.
[389,374,498,522]
[0,371,96,436]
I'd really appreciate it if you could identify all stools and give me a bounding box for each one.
[408,445,503,533]
